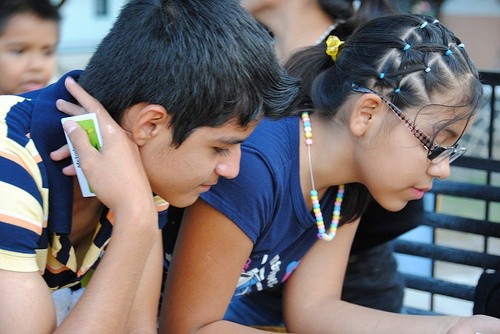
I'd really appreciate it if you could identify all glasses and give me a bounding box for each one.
[350,83,468,164]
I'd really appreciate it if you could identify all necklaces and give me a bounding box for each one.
[300,112,345,240]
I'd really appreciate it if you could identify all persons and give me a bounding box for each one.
[2,0,301,334]
[238,0,397,113]
[147,11,500,334]
[0,0,60,92]
